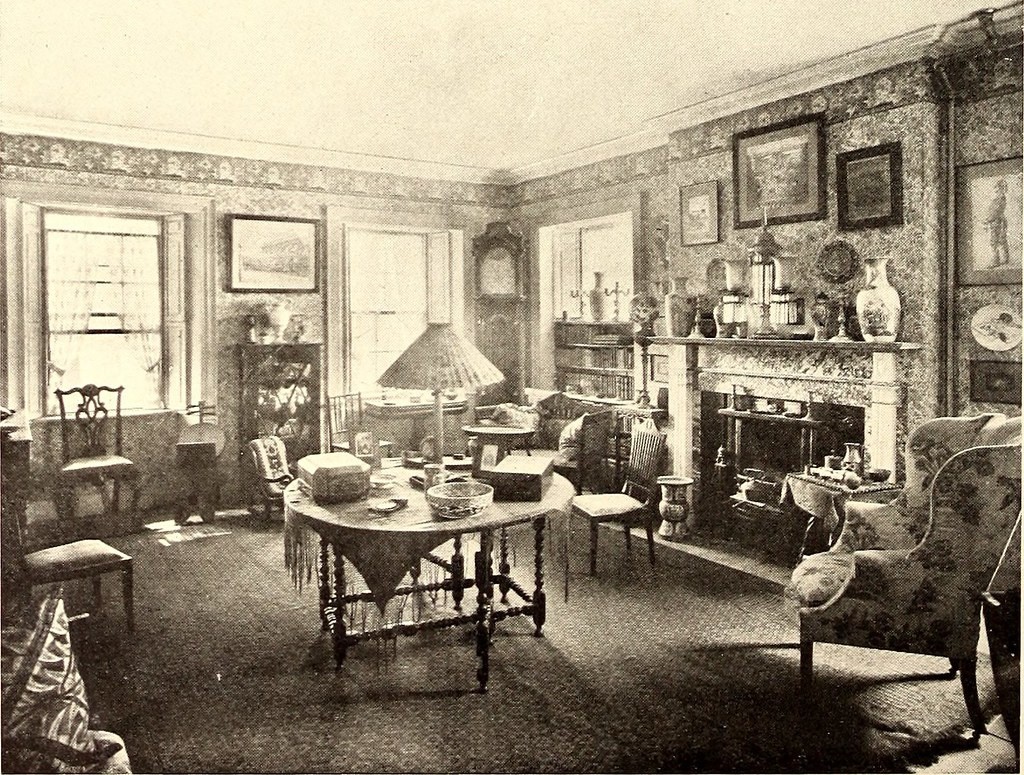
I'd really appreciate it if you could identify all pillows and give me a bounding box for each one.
[560,411,591,462]
[1,580,123,774]
[493,404,544,451]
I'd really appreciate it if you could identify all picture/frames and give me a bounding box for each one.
[226,213,320,295]
[678,180,719,247]
[955,157,1022,286]
[732,112,829,229]
[834,140,904,231]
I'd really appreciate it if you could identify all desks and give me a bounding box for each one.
[283,399,576,696]
[32,410,188,517]
[778,473,903,559]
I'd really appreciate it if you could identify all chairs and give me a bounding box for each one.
[795,411,1021,735]
[0,383,140,641]
[570,428,670,577]
[244,393,393,525]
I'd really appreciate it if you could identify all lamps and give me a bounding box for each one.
[374,320,506,489]
[747,223,785,342]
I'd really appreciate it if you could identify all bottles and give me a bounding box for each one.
[665,276,697,338]
[856,256,901,342]
[589,272,607,321]
[841,441,863,476]
[656,473,694,540]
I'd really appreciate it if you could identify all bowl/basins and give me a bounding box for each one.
[867,469,892,482]
[426,482,495,518]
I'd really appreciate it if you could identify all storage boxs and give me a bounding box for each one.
[491,456,555,501]
[294,454,371,503]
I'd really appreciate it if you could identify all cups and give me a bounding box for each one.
[824,456,842,470]
[424,463,445,499]
[368,473,396,509]
[805,464,817,476]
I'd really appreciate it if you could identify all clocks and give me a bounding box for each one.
[469,220,531,404]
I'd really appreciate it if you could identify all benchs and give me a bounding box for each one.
[474,390,612,497]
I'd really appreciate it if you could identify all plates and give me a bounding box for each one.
[970,304,1022,352]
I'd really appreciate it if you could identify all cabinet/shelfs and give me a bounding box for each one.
[238,341,324,499]
[1,410,34,606]
[554,321,635,409]
